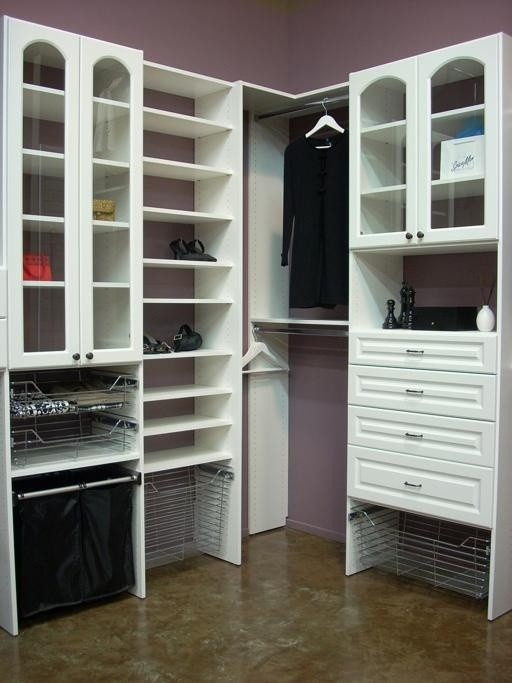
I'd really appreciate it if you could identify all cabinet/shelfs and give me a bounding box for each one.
[2,15,140,478]
[141,58,241,474]
[348,34,502,528]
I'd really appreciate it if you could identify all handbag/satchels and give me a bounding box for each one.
[143,323,203,354]
[92,198,116,221]
[23,252,52,281]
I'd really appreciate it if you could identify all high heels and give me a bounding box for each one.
[169,238,218,262]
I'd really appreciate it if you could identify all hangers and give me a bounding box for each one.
[241,324,293,376]
[282,97,348,159]
